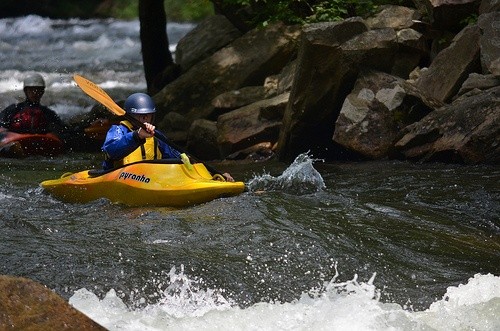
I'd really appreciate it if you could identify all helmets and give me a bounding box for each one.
[23,74,46,90]
[123,94,157,114]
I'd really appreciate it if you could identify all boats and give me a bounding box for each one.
[39,160,245,207]
[0,126,62,157]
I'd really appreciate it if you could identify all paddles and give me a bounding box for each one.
[0,118,110,140]
[73,74,266,194]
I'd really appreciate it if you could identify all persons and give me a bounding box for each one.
[0,74,67,144]
[101,92,235,183]
